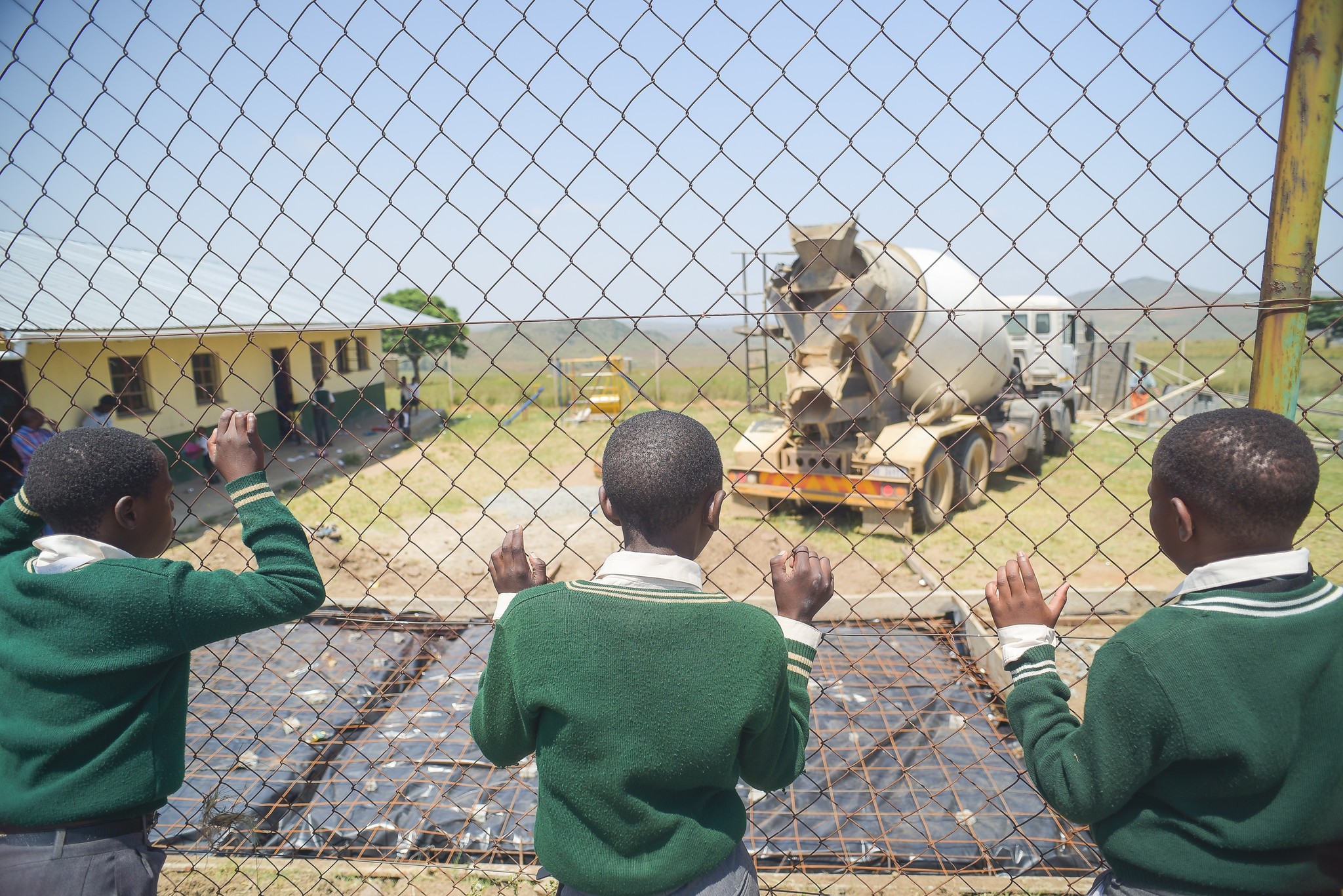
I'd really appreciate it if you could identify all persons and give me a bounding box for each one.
[11,407,61,473]
[194,426,221,484]
[1130,363,1163,424]
[398,403,411,441]
[1,408,326,896]
[287,403,303,445]
[310,377,336,447]
[469,410,835,896]
[387,408,398,425]
[399,377,413,413]
[409,378,420,415]
[81,394,116,427]
[984,407,1343,896]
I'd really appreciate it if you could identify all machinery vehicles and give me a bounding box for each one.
[721,212,1095,540]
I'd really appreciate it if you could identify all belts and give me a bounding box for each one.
[0,810,160,847]
